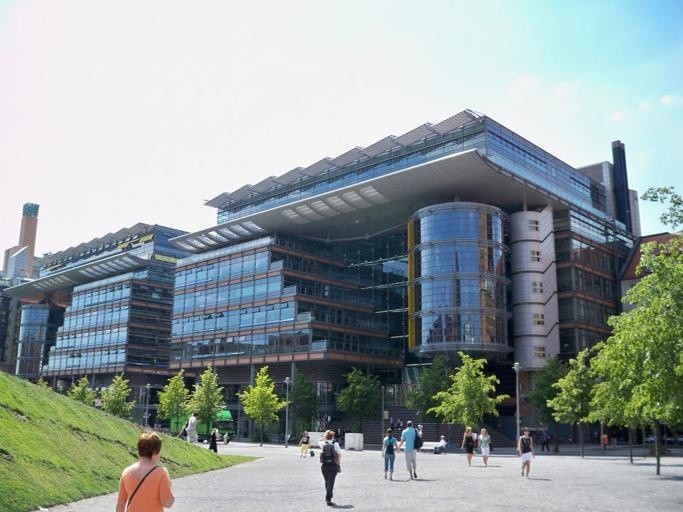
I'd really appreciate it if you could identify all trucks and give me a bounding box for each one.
[170,404,235,445]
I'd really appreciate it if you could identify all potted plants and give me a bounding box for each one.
[344,421,364,450]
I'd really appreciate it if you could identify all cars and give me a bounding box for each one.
[643,434,682,445]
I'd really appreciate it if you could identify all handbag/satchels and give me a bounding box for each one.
[414,435,422,449]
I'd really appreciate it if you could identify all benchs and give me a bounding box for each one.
[394,441,406,451]
[420,441,447,454]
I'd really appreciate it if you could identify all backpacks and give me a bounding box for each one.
[387,439,395,454]
[320,442,336,465]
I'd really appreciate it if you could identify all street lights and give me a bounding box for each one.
[283,376,291,447]
[145,383,152,426]
[512,362,522,455]
[50,344,57,391]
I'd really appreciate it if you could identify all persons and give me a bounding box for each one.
[478,427,491,467]
[299,430,310,458]
[434,435,446,454]
[116,432,175,512]
[187,412,199,445]
[208,428,218,454]
[517,427,533,479]
[399,421,423,481]
[609,428,617,449]
[461,426,474,466]
[381,430,399,479]
[388,414,412,429]
[318,429,341,504]
[541,430,551,452]
